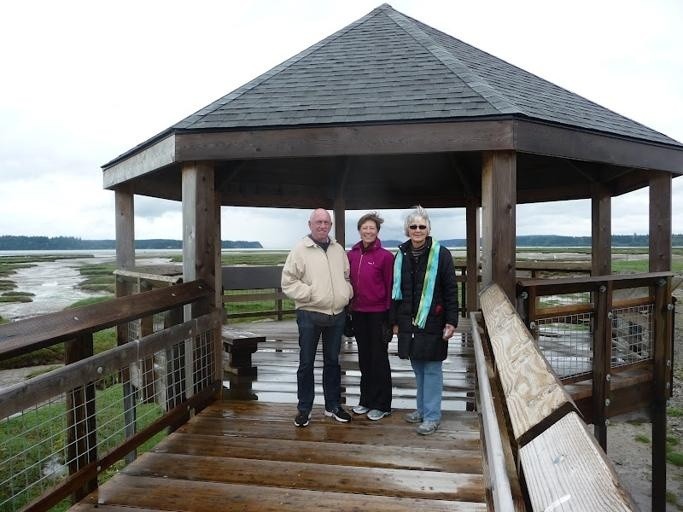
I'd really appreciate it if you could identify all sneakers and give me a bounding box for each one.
[322,405,352,423]
[293,407,312,427]
[415,418,441,435]
[365,406,392,421]
[402,409,423,424]
[351,402,370,415]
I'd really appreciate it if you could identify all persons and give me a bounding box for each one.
[280,208,354,427]
[346,213,396,420]
[389,205,459,435]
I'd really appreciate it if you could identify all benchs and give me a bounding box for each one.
[223,325,266,392]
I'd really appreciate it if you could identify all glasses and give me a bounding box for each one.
[408,224,427,230]
